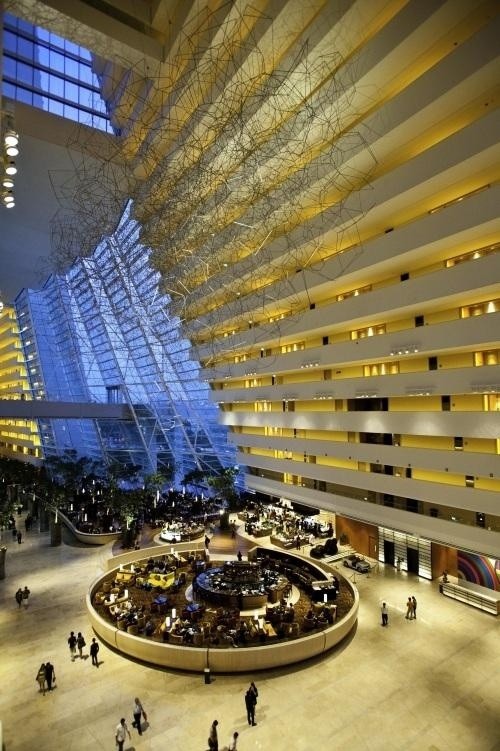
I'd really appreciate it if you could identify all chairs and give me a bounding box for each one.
[96,550,341,649]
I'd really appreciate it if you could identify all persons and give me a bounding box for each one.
[405,597,414,620]
[44,661,56,693]
[207,680,258,751]
[68,631,100,668]
[381,602,388,626]
[115,718,132,751]
[1,455,342,610]
[412,596,417,620]
[131,697,148,736]
[36,663,47,696]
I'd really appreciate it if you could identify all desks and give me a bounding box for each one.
[237,506,334,548]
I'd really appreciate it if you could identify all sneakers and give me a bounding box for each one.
[252,722,257,726]
[249,720,251,724]
